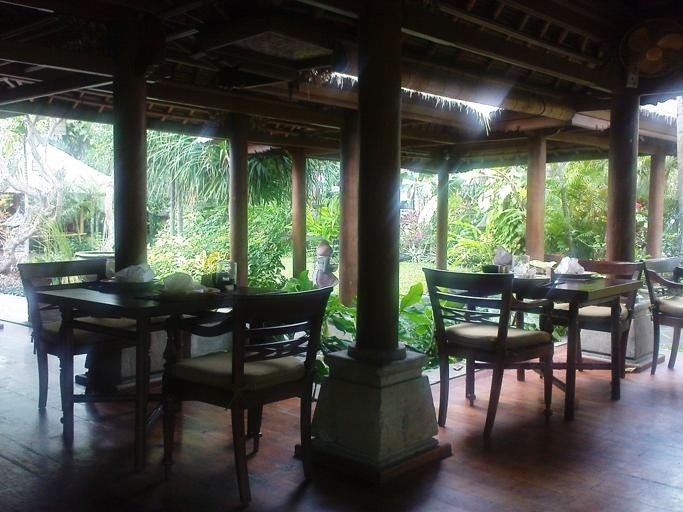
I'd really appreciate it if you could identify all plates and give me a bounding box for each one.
[553,270,597,280]
[98,277,160,294]
[512,273,550,284]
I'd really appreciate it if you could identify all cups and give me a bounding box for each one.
[512,254,530,273]
[214,260,237,287]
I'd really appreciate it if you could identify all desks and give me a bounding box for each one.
[465,275,642,420]
[32,285,289,459]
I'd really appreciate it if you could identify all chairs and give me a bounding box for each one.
[17,258,171,425]
[642,258,683,374]
[162,285,332,504]
[423,266,556,438]
[540,258,646,381]
